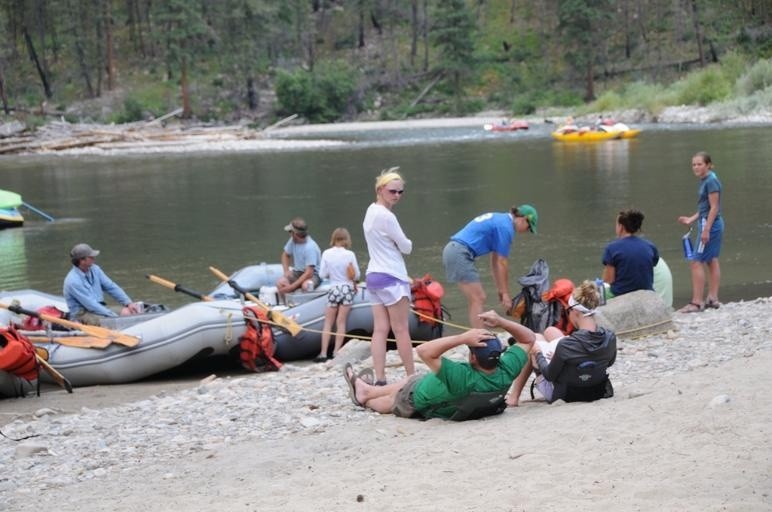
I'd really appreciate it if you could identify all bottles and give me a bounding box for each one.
[307,281,314,292]
[698,239,705,254]
[682,225,694,258]
[137,301,144,314]
[595,277,606,306]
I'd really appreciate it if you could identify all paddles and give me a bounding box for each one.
[1,303,141,350]
[145,267,303,339]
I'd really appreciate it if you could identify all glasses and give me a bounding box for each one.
[383,189,403,194]
[556,303,563,315]
[565,302,580,315]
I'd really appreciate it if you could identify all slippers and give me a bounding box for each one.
[358,369,374,408]
[342,360,363,407]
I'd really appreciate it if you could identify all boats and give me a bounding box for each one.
[550,128,644,141]
[213,260,448,361]
[0,279,258,392]
[0,204,27,229]
[482,122,531,132]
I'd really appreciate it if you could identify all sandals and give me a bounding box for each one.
[679,302,705,315]
[704,298,721,311]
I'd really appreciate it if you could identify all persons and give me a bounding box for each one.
[602,210,660,299]
[362,166,417,386]
[314,227,361,362]
[63,243,142,328]
[677,149,725,314]
[344,309,537,419]
[505,281,616,408]
[441,204,537,329]
[276,217,321,305]
[558,115,615,131]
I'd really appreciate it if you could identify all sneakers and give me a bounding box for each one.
[313,356,327,363]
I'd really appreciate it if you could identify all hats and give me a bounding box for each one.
[467,334,503,360]
[69,243,100,261]
[284,222,308,234]
[515,204,538,234]
